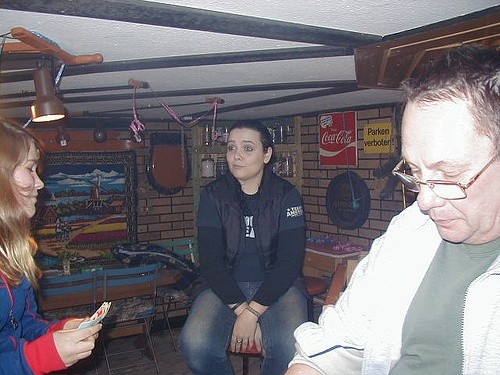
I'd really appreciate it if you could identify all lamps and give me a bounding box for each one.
[31,66,67,123]
[130,130,143,143]
[56,126,70,143]
[93,122,107,142]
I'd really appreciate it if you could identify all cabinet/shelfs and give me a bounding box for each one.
[191,117,303,234]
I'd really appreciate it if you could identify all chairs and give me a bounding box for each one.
[40,262,180,375]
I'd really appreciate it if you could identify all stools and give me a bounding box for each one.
[303,276,327,321]
[227,342,261,375]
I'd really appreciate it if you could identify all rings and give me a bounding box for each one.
[237,341,242,343]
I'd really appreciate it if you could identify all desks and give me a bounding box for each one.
[305,247,368,286]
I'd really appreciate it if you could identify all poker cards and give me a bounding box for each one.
[77,301,112,330]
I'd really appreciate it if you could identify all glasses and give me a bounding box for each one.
[392,154,498,200]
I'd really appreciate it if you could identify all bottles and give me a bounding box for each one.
[202,124,293,178]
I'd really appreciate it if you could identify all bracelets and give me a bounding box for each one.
[231,298,245,311]
[246,306,261,317]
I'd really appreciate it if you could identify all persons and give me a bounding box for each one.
[284,43,500,375]
[0,115,102,375]
[177,120,313,375]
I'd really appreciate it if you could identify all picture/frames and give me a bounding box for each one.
[31,151,138,270]
